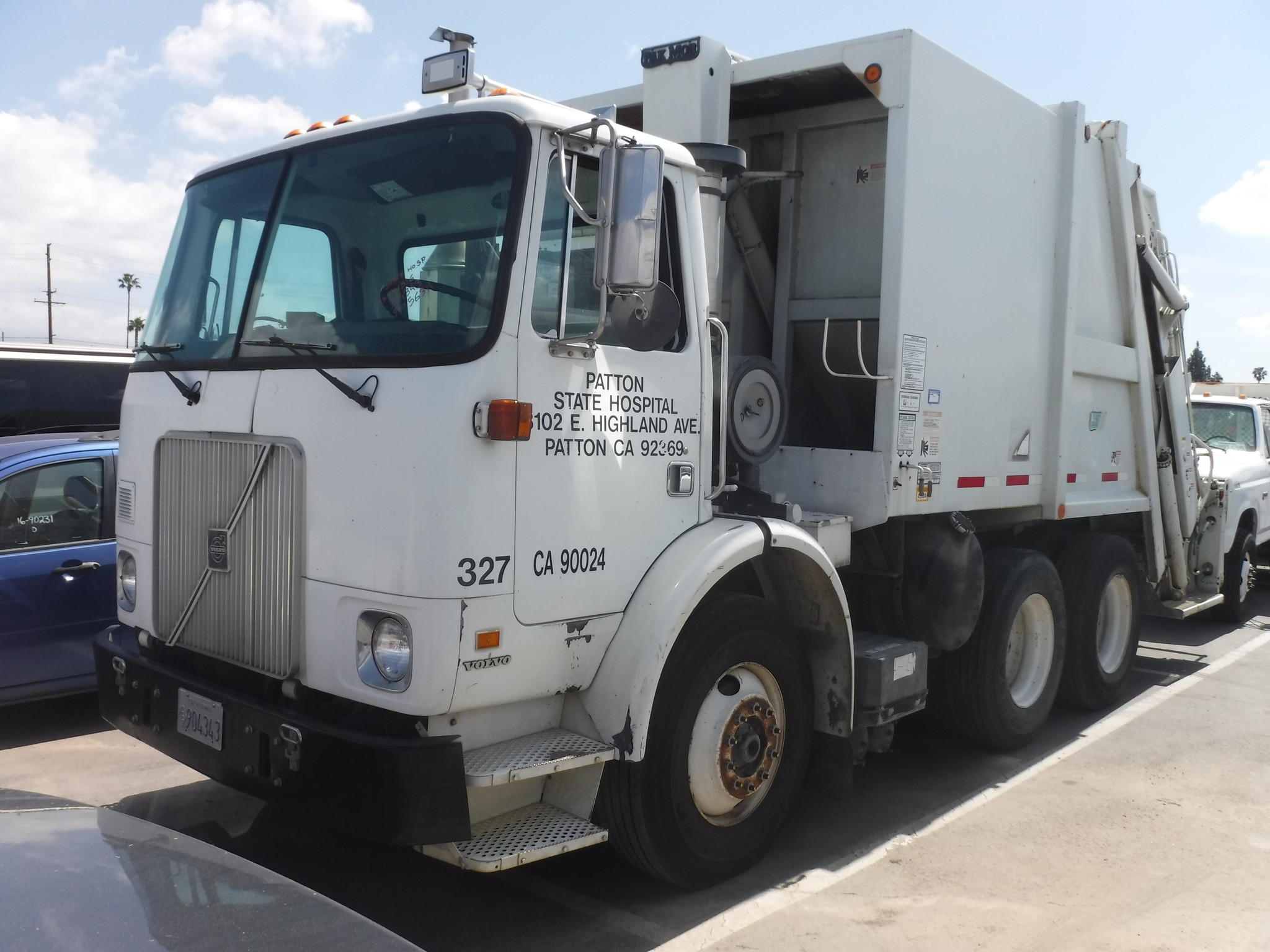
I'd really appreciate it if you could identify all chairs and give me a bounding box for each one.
[34,475,102,546]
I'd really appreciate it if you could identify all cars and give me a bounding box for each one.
[0,425,137,729]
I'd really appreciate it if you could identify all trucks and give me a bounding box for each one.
[1177,381,1270,625]
[88,22,1227,881]
